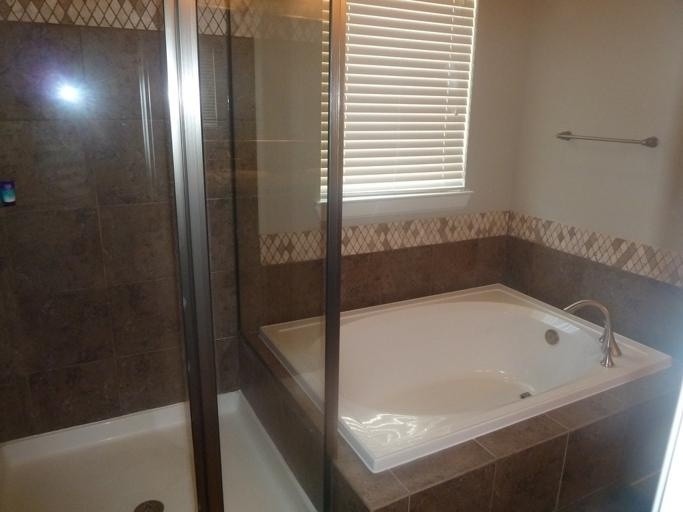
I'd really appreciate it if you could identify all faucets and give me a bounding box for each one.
[562,298,613,354]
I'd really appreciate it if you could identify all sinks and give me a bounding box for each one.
[306,299,603,419]
